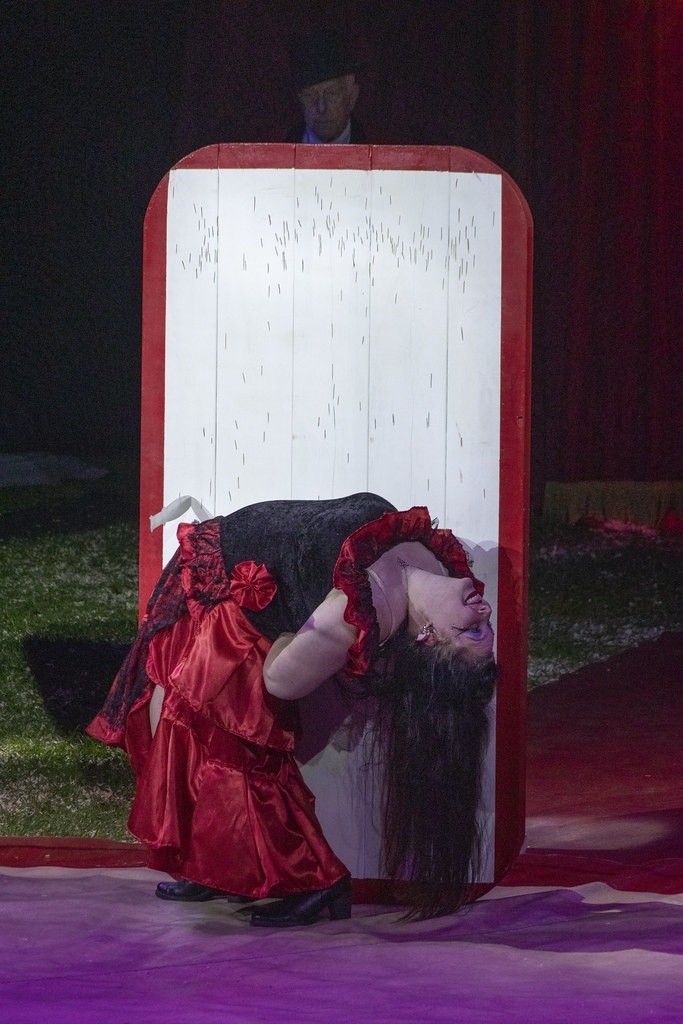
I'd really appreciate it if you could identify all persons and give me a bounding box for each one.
[262,33,412,144]
[85,492,499,928]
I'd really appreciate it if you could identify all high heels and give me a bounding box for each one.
[155,878,249,903]
[250,873,352,928]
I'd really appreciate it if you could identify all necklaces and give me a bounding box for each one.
[396,557,423,613]
